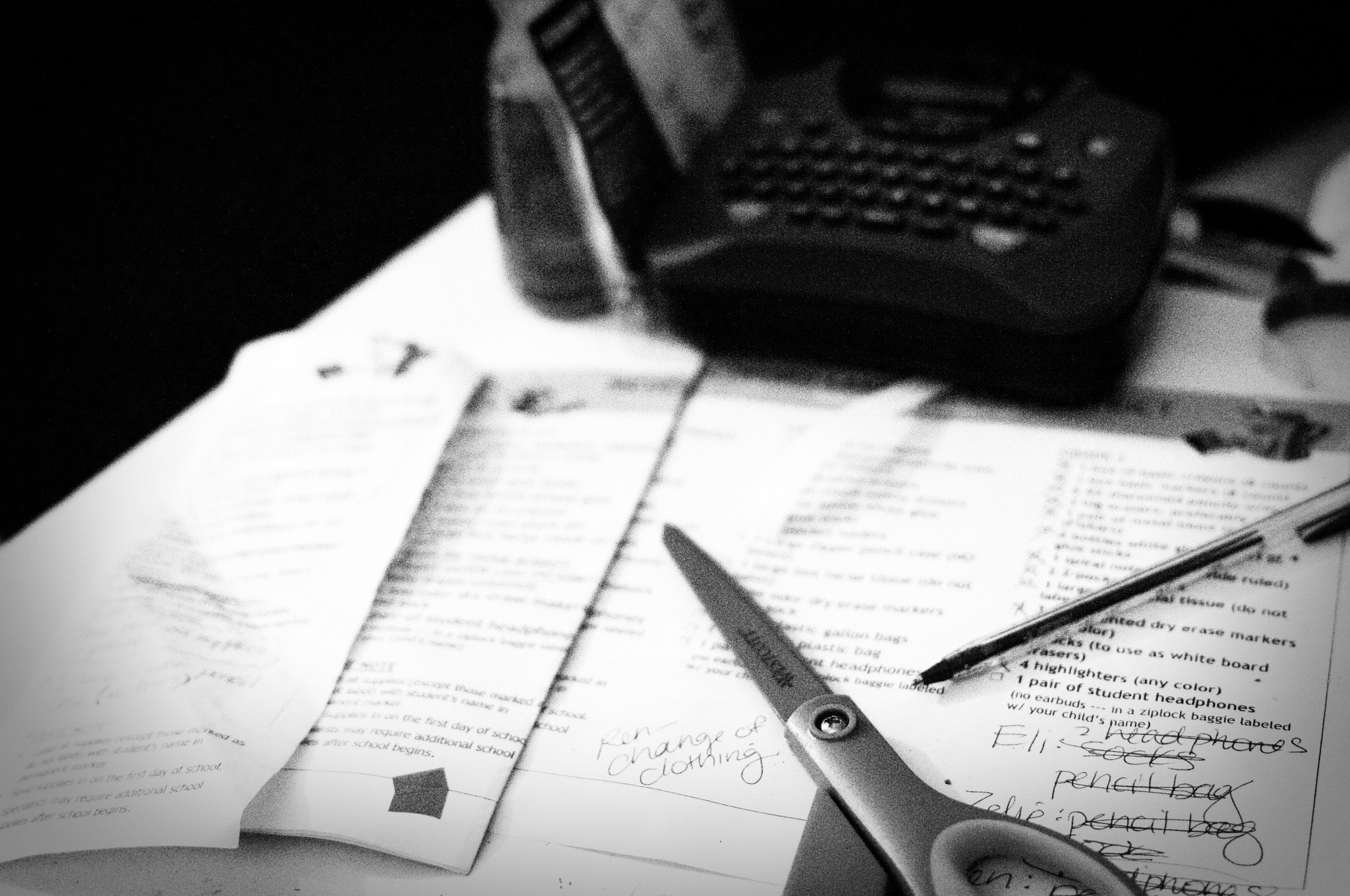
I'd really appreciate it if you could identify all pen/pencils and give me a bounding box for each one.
[911,477,1350,686]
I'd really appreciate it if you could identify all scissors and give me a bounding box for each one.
[661,522,1148,896]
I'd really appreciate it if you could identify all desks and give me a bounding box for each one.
[0,127,1350,895]
